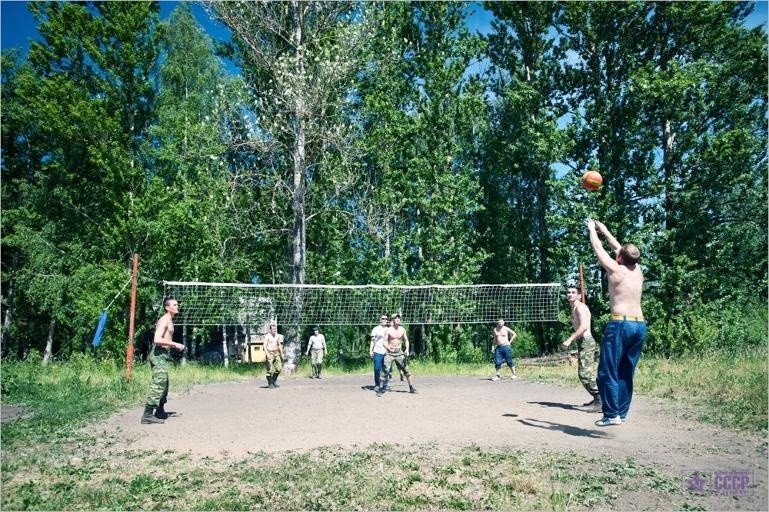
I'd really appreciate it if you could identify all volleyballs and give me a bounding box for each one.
[582,171,603,190]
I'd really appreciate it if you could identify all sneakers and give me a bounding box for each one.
[410,387,417,393]
[584,394,603,413]
[594,415,622,426]
[154,412,168,420]
[373,385,383,397]
[510,374,517,380]
[269,382,280,389]
[141,413,164,425]
[400,375,405,381]
[492,374,501,381]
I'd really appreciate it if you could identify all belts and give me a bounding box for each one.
[609,313,645,321]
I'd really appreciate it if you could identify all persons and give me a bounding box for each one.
[377,313,420,395]
[557,286,607,412]
[140,297,188,424]
[582,216,648,426]
[387,316,406,382]
[262,325,286,387]
[305,326,329,378]
[368,314,393,392]
[489,318,519,382]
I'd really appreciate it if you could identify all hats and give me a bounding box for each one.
[390,313,402,319]
[312,327,319,331]
[380,315,388,319]
